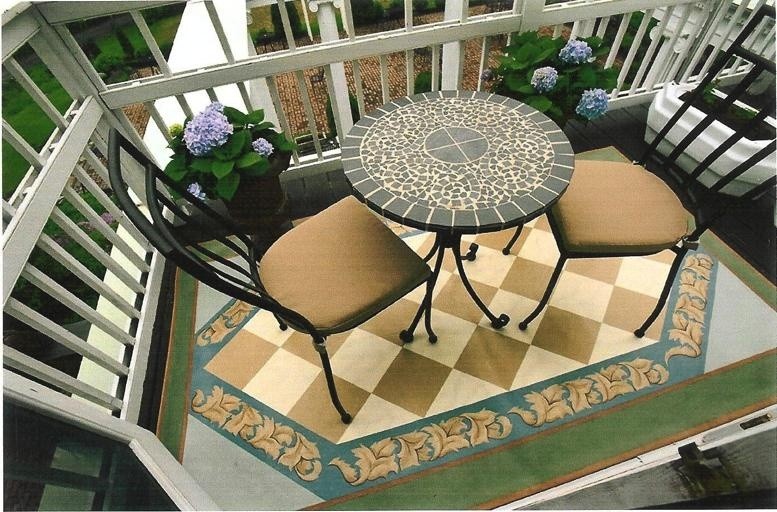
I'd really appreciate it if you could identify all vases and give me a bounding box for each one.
[219,174,289,227]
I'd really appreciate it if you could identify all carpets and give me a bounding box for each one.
[155,139,776,510]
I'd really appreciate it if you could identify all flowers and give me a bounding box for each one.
[162,100,299,201]
[480,27,621,127]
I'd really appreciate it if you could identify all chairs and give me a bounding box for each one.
[108,126,437,425]
[500,4,776,339]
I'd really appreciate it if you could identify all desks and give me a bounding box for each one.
[340,88,575,345]
